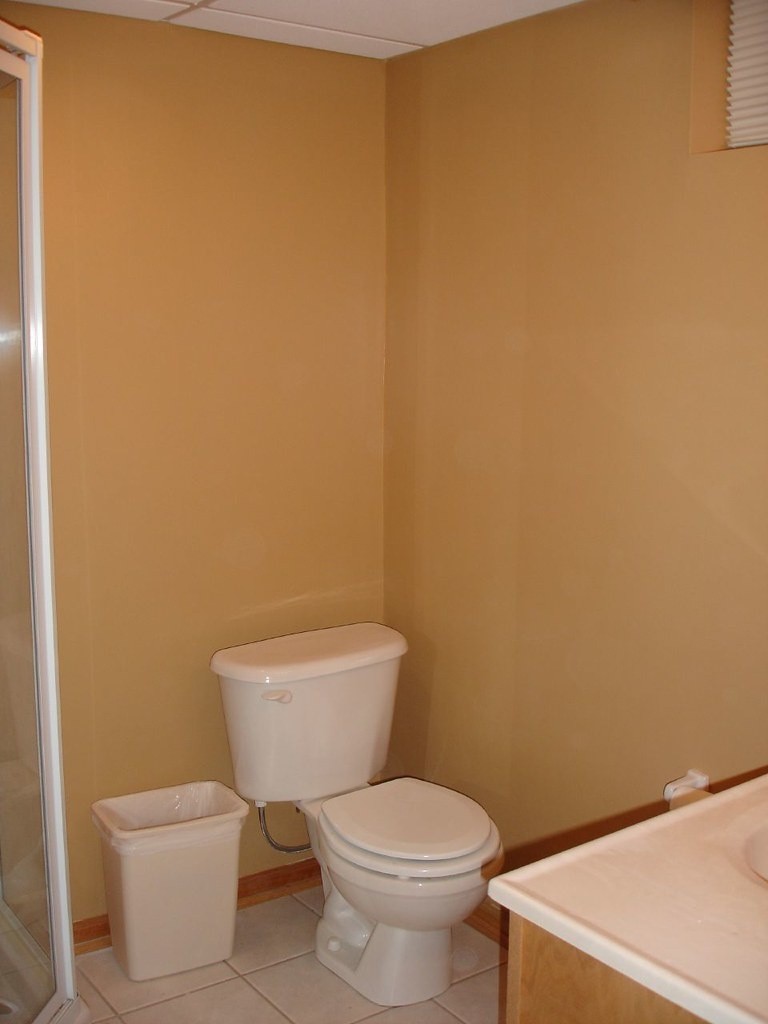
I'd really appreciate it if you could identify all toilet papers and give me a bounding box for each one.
[669,785,714,811]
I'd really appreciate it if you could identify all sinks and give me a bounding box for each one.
[722,799,768,890]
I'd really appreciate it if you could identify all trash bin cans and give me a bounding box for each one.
[90,781,250,983]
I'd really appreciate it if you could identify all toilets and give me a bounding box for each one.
[209,621,505,1007]
